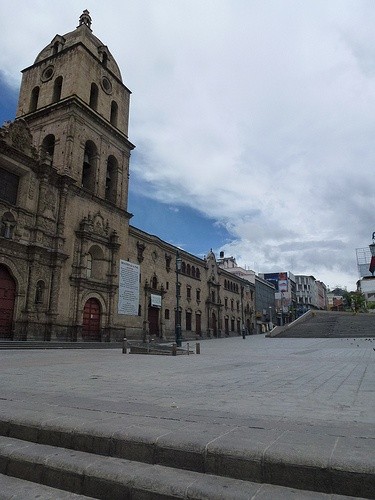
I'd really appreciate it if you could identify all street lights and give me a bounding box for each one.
[173,250,183,345]
[240,287,246,339]
